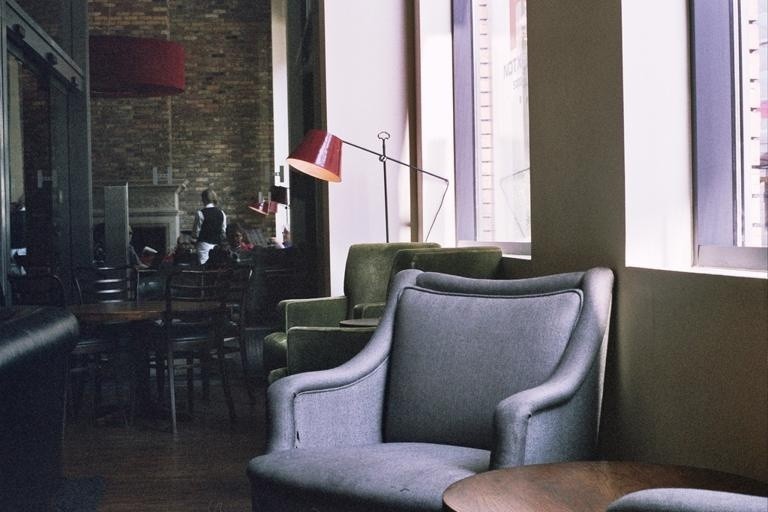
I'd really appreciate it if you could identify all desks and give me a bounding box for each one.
[443,460,768,511]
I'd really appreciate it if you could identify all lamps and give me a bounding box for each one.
[246,199,278,216]
[286,128,447,244]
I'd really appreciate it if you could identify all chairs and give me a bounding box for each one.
[264,244,503,380]
[246,267,614,510]
[11,264,253,433]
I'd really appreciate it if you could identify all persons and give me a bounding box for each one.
[219,224,254,257]
[191,190,226,266]
[129,226,142,264]
